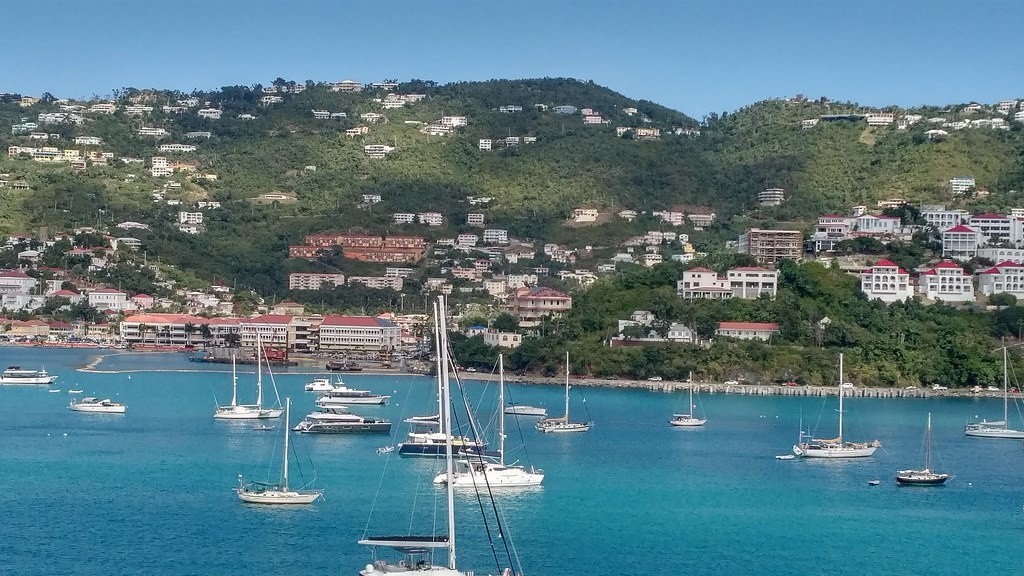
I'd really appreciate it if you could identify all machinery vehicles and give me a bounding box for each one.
[212,276,286,362]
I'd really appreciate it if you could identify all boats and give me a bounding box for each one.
[304,378,334,391]
[0,366,59,384]
[291,406,392,435]
[868,480,880,486]
[314,373,392,409]
[69,397,127,413]
[253,424,276,430]
[325,355,364,373]
[775,455,795,460]
[505,405,546,417]
[376,445,394,454]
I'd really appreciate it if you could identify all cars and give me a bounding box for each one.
[307,336,527,377]
[781,382,798,387]
[1007,387,1021,394]
[604,375,618,380]
[839,383,853,389]
[970,385,984,394]
[724,380,739,385]
[985,385,1000,391]
[0,334,137,351]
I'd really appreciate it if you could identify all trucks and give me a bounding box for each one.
[647,376,662,382]
[933,383,948,391]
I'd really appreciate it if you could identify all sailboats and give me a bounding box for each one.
[399,307,501,462]
[236,397,323,505]
[355,295,531,576]
[668,371,709,426]
[212,333,285,421]
[965,338,1024,440]
[894,412,951,486]
[535,350,595,435]
[792,352,881,458]
[431,354,548,491]
